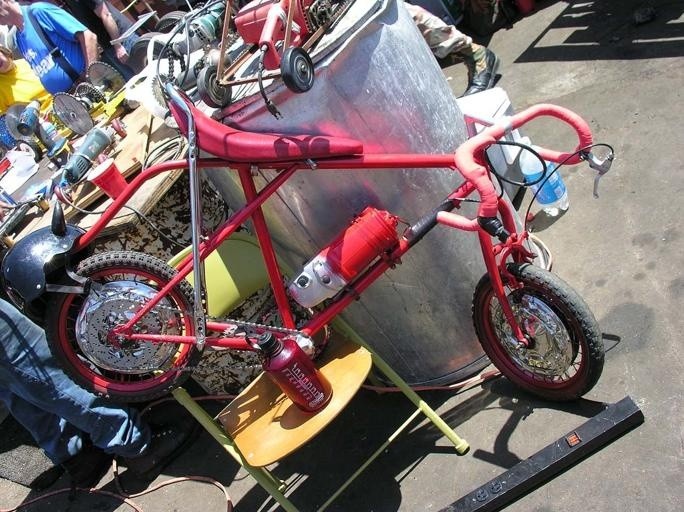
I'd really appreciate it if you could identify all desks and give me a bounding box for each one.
[0,44,190,239]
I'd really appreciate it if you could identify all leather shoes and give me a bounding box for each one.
[124,414,202,482]
[464,45,500,96]
[71,449,105,487]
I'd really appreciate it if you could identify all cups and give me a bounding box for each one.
[86,157,129,203]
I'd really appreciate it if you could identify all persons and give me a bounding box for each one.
[403,2,501,97]
[0,44,54,116]
[0,299,201,480]
[0,0,126,99]
[62,0,148,81]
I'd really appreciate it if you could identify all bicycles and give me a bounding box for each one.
[44,80,615,404]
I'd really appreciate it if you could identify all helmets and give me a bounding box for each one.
[1,201,87,303]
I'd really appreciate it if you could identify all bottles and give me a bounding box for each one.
[244,325,333,413]
[520,135,570,217]
[288,205,400,310]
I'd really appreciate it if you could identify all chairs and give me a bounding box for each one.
[143,235,469,511]
